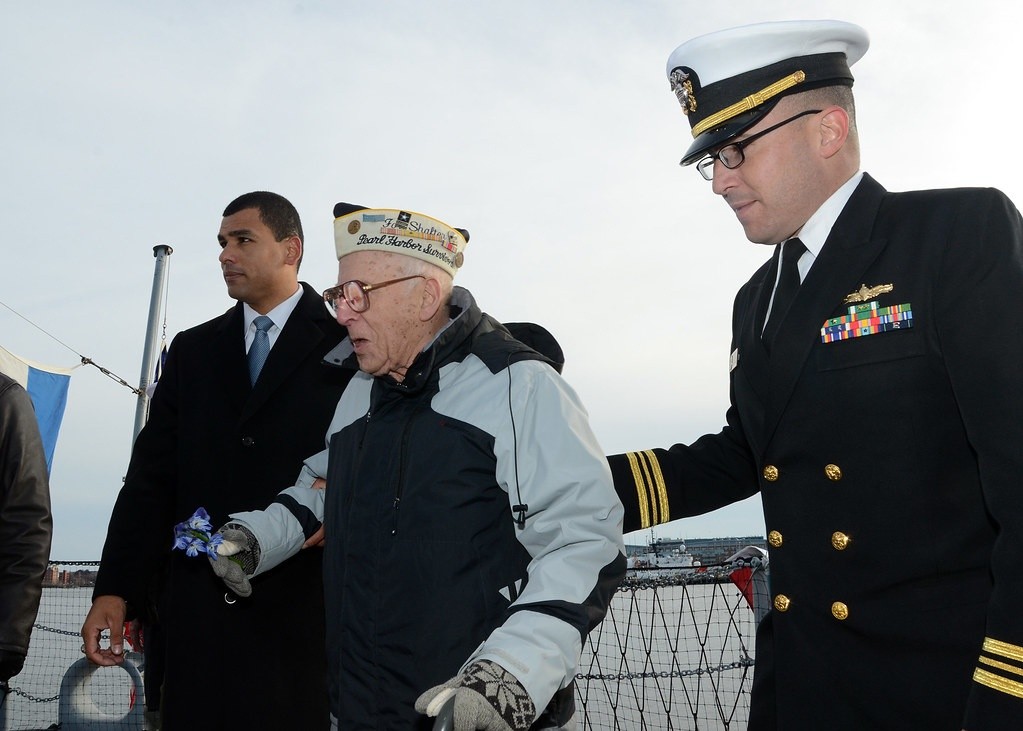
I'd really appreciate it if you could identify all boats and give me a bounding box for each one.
[623,527,702,582]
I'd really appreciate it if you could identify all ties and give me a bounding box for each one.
[246,316,275,389]
[762,237,808,358]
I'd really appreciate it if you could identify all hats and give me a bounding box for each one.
[333,203,470,280]
[667,19,870,166]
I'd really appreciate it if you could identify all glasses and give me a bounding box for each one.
[696,110,821,181]
[323,276,426,320]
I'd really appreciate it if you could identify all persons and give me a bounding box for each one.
[81,191,361,731]
[0,372,53,682]
[606,21,1023,731]
[208,203,628,731]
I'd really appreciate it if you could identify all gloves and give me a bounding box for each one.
[415,660,535,731]
[207,524,261,596]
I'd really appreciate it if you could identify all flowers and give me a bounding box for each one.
[170,506,248,571]
